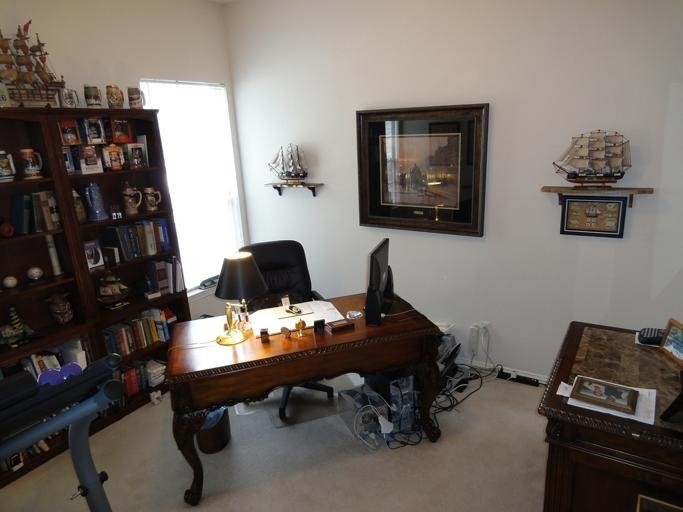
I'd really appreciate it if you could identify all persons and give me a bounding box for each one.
[583,379,628,402]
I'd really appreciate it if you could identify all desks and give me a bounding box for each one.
[164,291,441,506]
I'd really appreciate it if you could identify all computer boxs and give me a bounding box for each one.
[373,368,419,443]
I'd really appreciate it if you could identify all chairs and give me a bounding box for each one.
[242,240,326,425]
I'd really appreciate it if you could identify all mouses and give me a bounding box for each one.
[285,304,301,314]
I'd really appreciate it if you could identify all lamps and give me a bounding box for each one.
[214,255,267,347]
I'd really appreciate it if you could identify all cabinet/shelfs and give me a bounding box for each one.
[537,320,682,511]
[2,107,193,490]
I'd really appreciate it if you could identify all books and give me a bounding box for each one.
[12,192,54,234]
[105,218,171,263]
[102,309,177,360]
[122,361,167,396]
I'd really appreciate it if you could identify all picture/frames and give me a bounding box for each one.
[658,318,683,367]
[356,103,490,238]
[570,375,640,414]
[560,196,628,239]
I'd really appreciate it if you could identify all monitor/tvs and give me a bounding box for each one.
[364,237,394,325]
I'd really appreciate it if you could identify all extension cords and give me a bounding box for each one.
[497,369,539,387]
